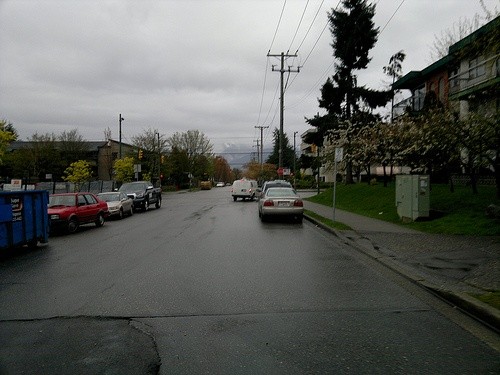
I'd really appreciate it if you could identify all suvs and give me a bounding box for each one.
[256,180,292,201]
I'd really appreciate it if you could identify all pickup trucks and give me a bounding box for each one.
[113,180,163,212]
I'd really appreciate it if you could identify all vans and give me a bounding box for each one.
[231,179,260,201]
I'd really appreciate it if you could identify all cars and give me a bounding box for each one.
[95,192,134,219]
[258,187,304,224]
[226,182,231,186]
[47,192,110,234]
[216,182,225,187]
